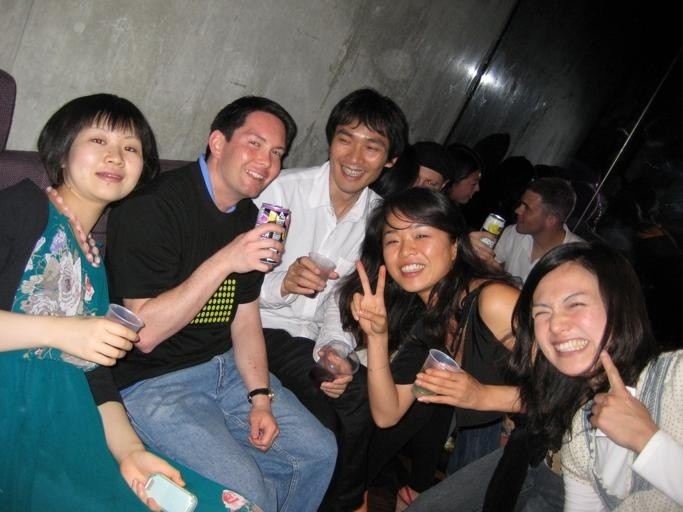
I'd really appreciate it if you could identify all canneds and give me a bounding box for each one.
[255,203,291,265]
[479,213,506,250]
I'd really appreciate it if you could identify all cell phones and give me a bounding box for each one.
[141,472,198,512]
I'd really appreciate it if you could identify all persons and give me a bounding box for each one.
[105,96,337,512]
[505,241,683,511]
[339,187,539,511]
[253,88,410,510]
[375,141,682,351]
[1,87,260,512]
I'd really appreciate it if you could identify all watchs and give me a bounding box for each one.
[248,388,274,404]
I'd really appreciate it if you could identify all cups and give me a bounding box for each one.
[305,251,337,299]
[106,304,145,335]
[411,349,462,404]
[308,341,361,389]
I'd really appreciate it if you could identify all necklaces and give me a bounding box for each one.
[47,185,100,267]
